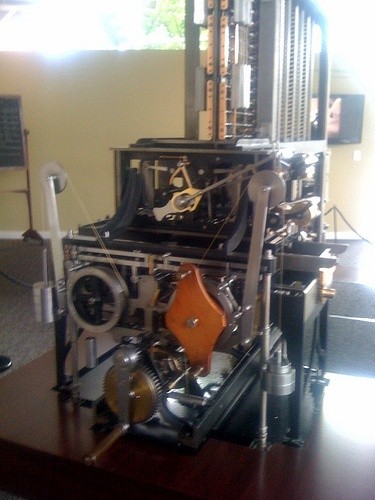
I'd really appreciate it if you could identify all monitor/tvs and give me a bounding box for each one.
[311,93,365,145]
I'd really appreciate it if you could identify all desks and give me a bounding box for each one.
[0,347,375,498]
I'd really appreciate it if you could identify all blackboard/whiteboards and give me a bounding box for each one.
[0,94,29,172]
